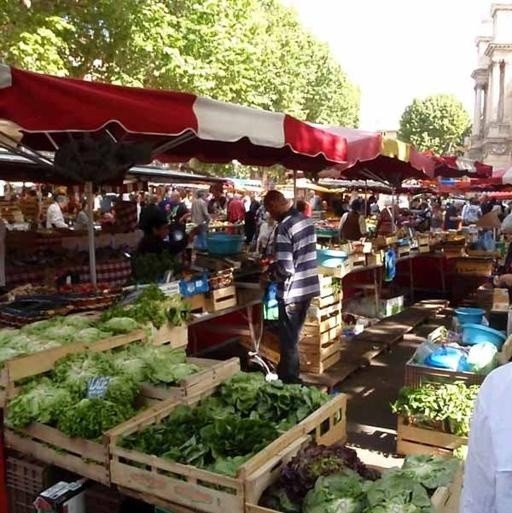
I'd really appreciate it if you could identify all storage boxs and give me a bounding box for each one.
[475,288,509,314]
[455,258,493,277]
[350,295,405,318]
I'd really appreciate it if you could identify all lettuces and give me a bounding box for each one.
[4,351,140,438]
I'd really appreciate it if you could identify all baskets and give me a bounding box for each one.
[404,348,487,391]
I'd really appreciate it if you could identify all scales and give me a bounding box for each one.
[194,252,257,272]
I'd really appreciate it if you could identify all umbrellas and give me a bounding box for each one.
[0,63,348,287]
[319,124,512,237]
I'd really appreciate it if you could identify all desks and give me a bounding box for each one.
[0,282,270,411]
[0,204,247,286]
[335,232,445,314]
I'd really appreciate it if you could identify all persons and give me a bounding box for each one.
[458,361,512,513]
[258,189,320,383]
[294,193,512,275]
[1,181,259,257]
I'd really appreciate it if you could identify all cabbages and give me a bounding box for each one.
[257,440,459,513]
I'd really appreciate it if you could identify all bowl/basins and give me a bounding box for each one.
[316,250,348,270]
[205,234,247,255]
[454,308,506,352]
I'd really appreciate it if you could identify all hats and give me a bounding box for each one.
[501,213,512,233]
